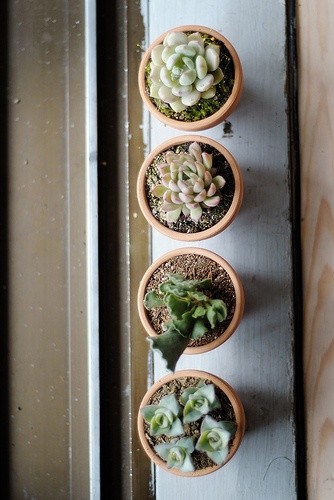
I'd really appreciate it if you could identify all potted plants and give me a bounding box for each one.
[136,246,246,375]
[135,134,244,242]
[137,370,247,478]
[137,24,245,131]
